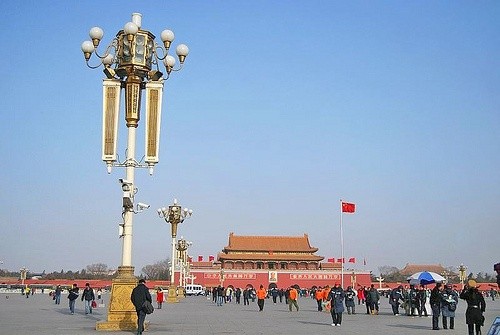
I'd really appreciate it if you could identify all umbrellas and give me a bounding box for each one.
[406,270,447,290]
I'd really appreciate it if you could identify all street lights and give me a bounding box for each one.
[440,270,449,285]
[20,267,28,284]
[81,11,194,331]
[457,264,468,290]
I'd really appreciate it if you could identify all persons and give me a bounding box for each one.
[458,279,487,335]
[81,282,96,315]
[269,273,276,282]
[204,284,500,318]
[326,282,344,328]
[438,284,458,330]
[429,283,450,330]
[66,283,80,314]
[20,283,102,304]
[154,286,167,310]
[130,279,153,335]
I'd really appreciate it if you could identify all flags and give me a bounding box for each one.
[337,258,344,263]
[349,258,355,263]
[187,257,192,262]
[342,202,355,213]
[328,258,334,262]
[198,256,204,262]
[208,255,214,262]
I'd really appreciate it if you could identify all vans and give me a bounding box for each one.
[176,285,202,296]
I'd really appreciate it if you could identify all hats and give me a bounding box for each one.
[139,279,146,283]
[467,279,476,289]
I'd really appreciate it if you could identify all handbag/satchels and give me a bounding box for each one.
[91,299,96,308]
[142,285,154,314]
